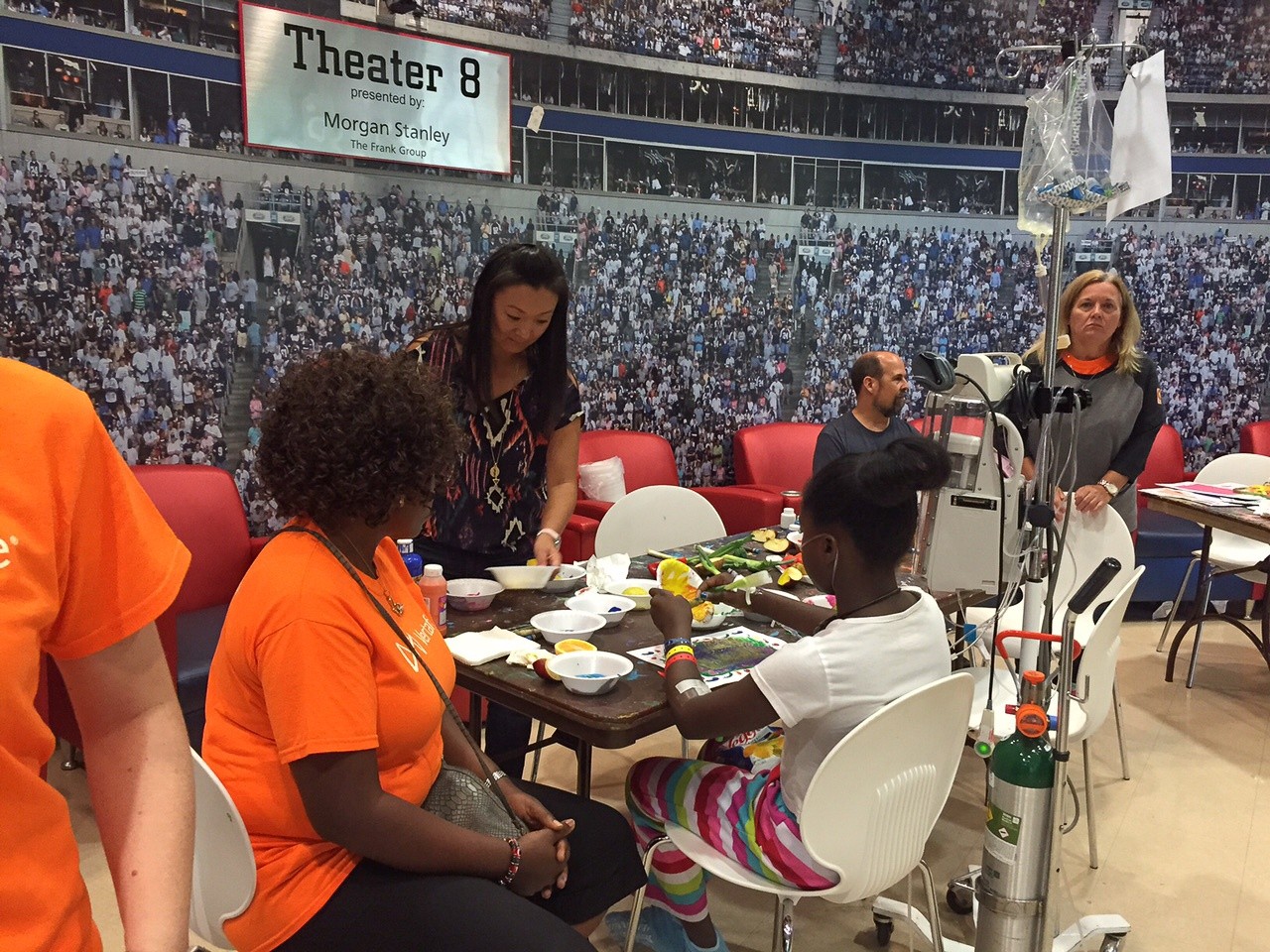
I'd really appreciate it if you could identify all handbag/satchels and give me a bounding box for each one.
[420,759,529,841]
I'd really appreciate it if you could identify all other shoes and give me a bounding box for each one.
[605,905,730,952]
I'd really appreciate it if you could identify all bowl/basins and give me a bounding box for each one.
[529,610,607,643]
[536,563,587,593]
[741,589,800,622]
[786,531,803,552]
[564,593,637,629]
[603,579,658,610]
[484,566,560,590]
[546,650,634,695]
[446,578,505,611]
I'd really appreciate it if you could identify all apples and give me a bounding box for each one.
[777,566,802,586]
[750,529,778,542]
[763,537,794,553]
[533,658,562,681]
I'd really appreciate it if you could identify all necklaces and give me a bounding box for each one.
[1067,352,1100,388]
[342,529,402,615]
[813,588,901,633]
[483,358,520,486]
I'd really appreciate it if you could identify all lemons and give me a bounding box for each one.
[622,587,648,595]
[554,638,597,654]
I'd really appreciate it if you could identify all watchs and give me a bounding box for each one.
[537,528,561,552]
[1097,480,1118,500]
[663,638,691,655]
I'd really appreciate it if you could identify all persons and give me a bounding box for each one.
[812,351,918,480]
[0,0,1270,533]
[0,358,195,952]
[1023,269,1163,533]
[605,434,955,952]
[202,348,648,952]
[397,242,585,780]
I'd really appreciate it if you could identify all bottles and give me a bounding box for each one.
[419,564,447,637]
[780,507,797,529]
[397,538,422,586]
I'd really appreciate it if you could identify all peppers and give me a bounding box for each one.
[647,557,688,578]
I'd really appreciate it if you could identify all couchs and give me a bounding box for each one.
[1128,421,1270,620]
[560,413,986,563]
[36,463,270,782]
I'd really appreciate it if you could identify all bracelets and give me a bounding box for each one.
[484,770,506,787]
[499,837,521,886]
[665,646,694,664]
[664,653,696,670]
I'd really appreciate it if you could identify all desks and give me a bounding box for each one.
[443,525,987,804]
[1139,487,1270,683]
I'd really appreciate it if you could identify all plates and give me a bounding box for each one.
[656,559,715,626]
[802,594,836,610]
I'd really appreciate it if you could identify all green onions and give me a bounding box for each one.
[646,533,801,608]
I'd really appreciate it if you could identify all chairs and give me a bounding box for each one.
[1155,453,1270,690]
[189,482,1146,952]
